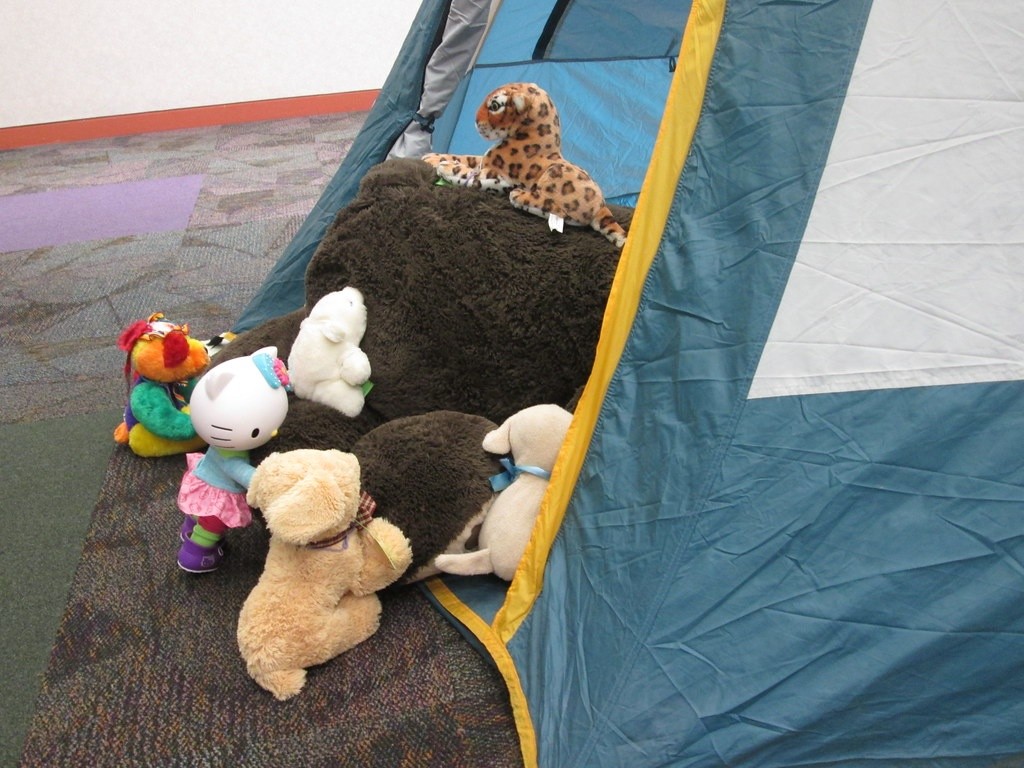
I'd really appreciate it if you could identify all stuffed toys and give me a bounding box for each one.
[114,84,638,700]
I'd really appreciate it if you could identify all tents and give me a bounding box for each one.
[232,0,1024,768]
